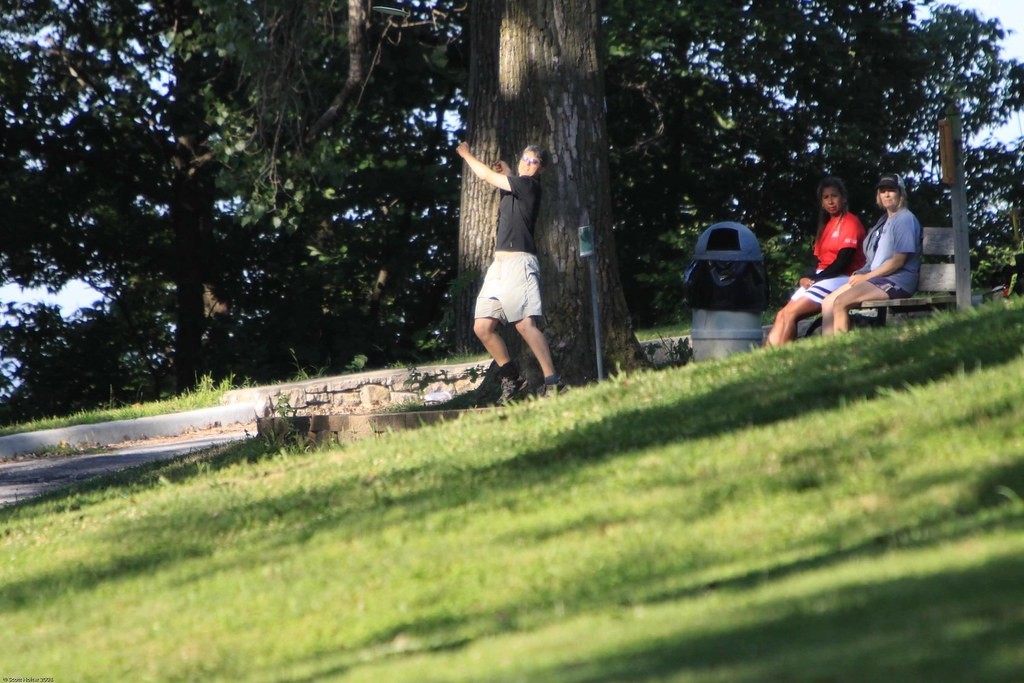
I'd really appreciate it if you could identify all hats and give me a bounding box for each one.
[876,174,898,188]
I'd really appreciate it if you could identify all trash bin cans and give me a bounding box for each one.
[685,221,768,363]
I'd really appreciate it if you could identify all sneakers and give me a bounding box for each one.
[498,374,529,403]
[541,376,568,400]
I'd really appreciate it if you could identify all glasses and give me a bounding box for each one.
[521,155,541,164]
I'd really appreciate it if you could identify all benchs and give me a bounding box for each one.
[803,225,955,340]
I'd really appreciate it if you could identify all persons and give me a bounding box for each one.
[762,177,869,347]
[821,175,922,336]
[454,140,571,406]
